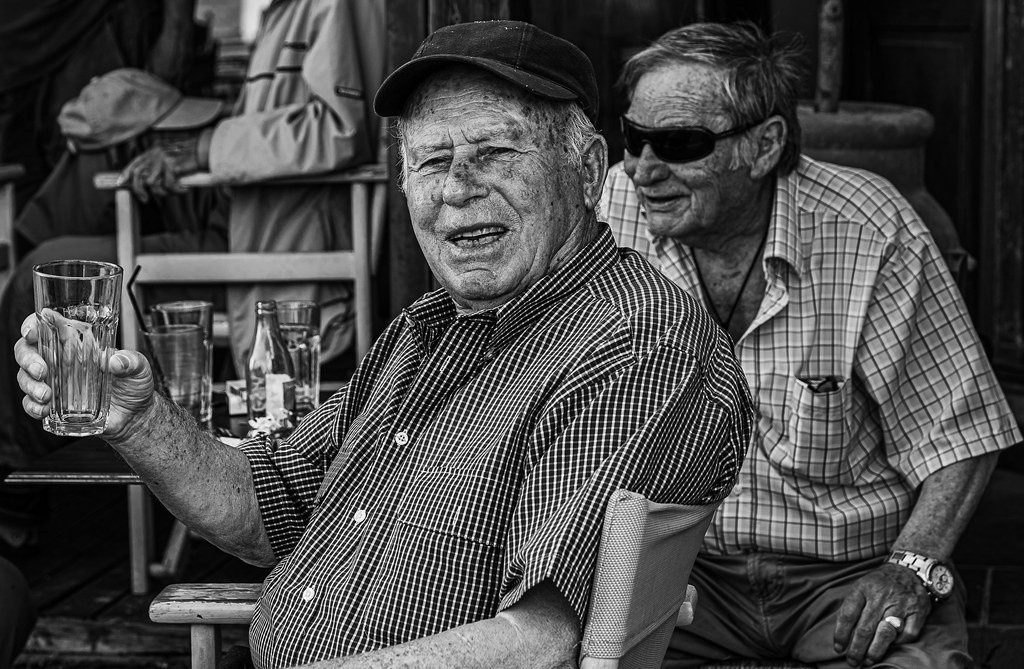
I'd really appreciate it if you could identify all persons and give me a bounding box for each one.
[15,21,753,669]
[0,0,365,350]
[591,22,1023,669]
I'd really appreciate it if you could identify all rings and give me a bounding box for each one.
[882,616,904,632]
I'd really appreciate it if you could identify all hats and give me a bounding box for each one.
[57,68,222,154]
[372,19,599,123]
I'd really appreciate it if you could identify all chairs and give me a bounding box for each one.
[144,489,721,669]
[92,30,395,399]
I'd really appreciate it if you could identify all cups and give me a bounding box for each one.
[139,323,206,423]
[32,259,123,437]
[275,300,323,422]
[155,300,215,423]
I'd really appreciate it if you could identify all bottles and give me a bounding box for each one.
[243,300,297,439]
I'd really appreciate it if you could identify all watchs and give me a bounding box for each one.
[883,551,954,602]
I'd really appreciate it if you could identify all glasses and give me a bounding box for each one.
[617,115,763,164]
